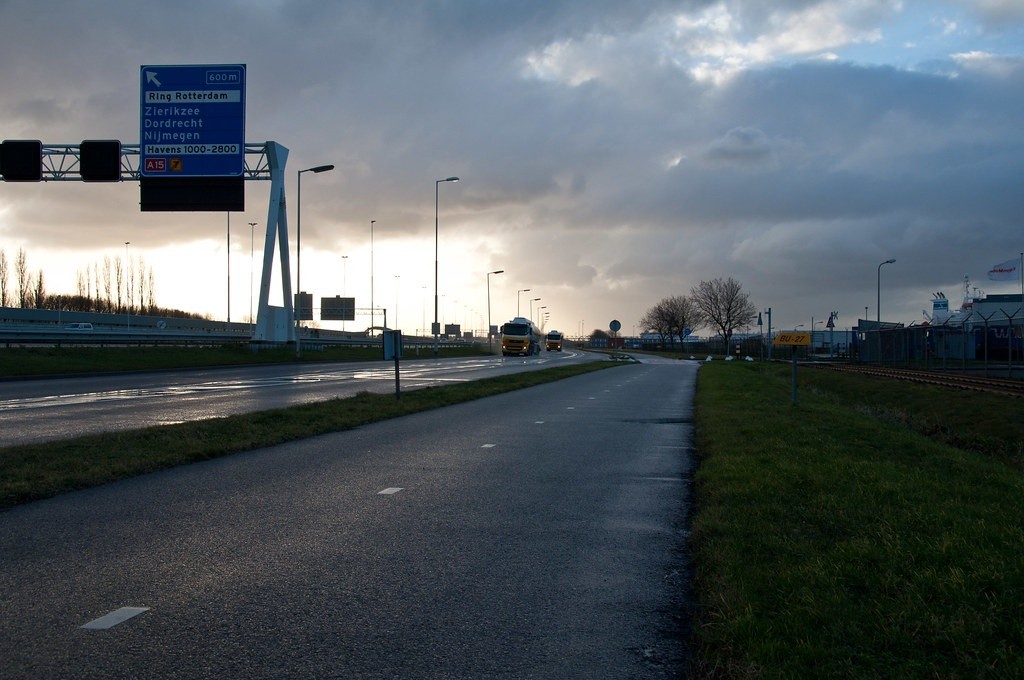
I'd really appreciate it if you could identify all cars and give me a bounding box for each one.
[64,323,93,331]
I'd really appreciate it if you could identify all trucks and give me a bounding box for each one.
[500,316,542,356]
[544,330,563,352]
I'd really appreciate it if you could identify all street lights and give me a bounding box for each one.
[541,312,550,330]
[746,316,757,358]
[441,294,445,334]
[249,222,258,334]
[530,297,541,321]
[877,258,896,329]
[342,255,349,338]
[371,219,376,338]
[422,286,427,337]
[487,270,504,353]
[537,307,547,327]
[434,176,460,354]
[395,275,400,329]
[517,289,530,318]
[814,321,823,355]
[464,304,484,337]
[124,241,131,332]
[794,324,804,332]
[297,165,334,354]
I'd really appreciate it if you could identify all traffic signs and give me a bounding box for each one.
[138,64,245,178]
